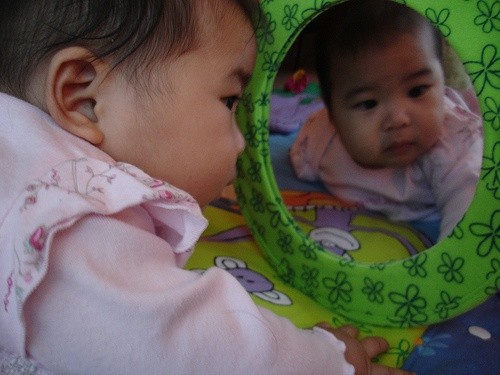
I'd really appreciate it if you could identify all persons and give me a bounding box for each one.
[294,0,482,243]
[0,0,416,375]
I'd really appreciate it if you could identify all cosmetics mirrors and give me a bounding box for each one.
[231,0,500,328]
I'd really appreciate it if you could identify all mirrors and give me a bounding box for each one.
[264,0,485,274]
